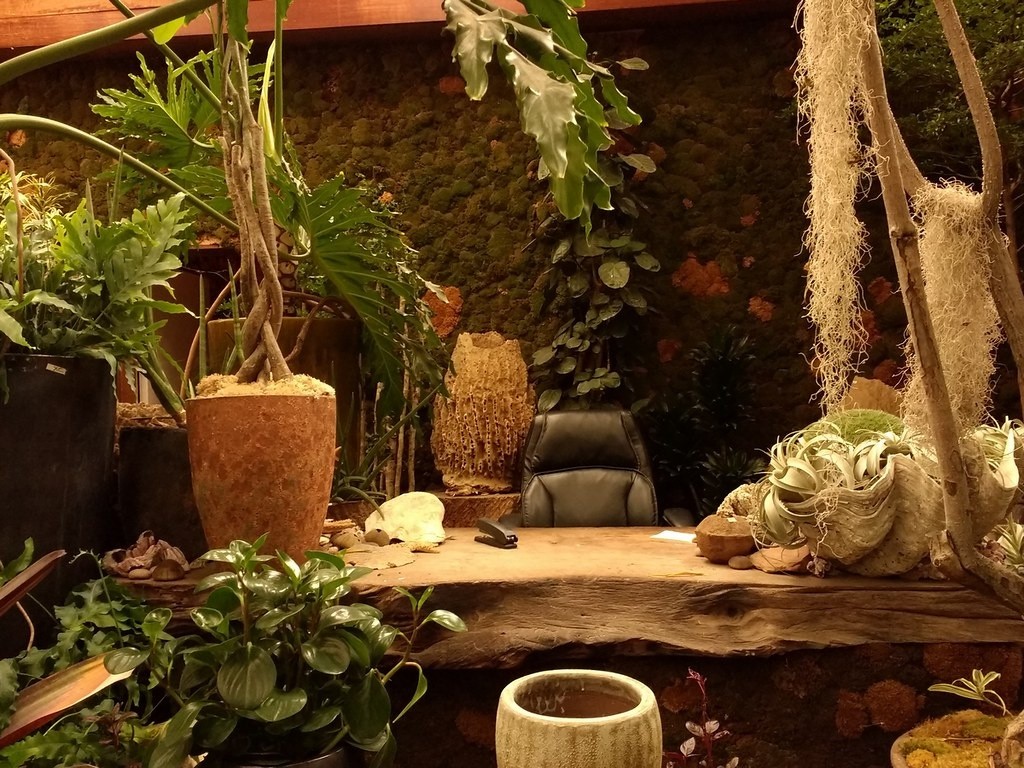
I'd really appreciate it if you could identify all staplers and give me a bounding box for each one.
[475,518,518,549]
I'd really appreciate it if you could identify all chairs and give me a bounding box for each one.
[520,408,659,528]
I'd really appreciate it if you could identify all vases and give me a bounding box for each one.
[496,668,663,768]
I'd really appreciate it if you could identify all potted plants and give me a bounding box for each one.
[0,0,661,768]
